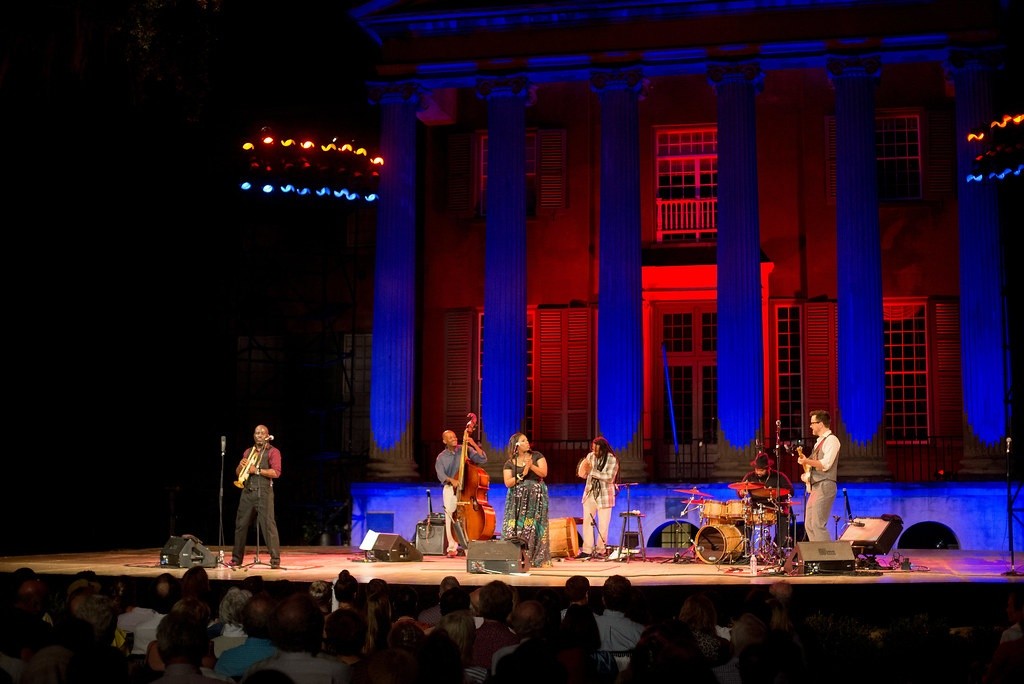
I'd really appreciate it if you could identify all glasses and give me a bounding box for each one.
[810,422,819,425]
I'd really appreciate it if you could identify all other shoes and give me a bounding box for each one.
[574,553,592,561]
[448,550,456,557]
[271,562,279,569]
[226,560,239,565]
[590,552,605,561]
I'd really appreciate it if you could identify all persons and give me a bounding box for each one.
[435,429,487,558]
[735,453,794,561]
[797,410,841,542]
[574,437,619,560]
[225,425,281,569]
[0,567,1024,684]
[503,431,554,568]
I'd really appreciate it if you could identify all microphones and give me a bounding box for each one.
[847,522,865,527]
[776,420,781,426]
[697,546,704,552]
[221,436,226,455]
[1005,437,1012,454]
[265,435,274,441]
[514,442,521,454]
[615,484,619,491]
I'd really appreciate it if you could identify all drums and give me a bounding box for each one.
[547,517,579,558]
[746,509,777,526]
[695,523,744,565]
[699,499,727,519]
[726,499,752,519]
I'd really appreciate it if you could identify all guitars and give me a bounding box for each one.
[795,446,812,493]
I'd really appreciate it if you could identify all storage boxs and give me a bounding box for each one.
[415,525,450,556]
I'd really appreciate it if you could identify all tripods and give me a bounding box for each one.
[582,513,607,562]
[717,485,794,572]
[660,515,698,564]
[217,440,288,572]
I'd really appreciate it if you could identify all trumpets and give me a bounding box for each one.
[234,446,258,489]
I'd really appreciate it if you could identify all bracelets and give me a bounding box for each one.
[255,465,261,475]
[517,473,523,480]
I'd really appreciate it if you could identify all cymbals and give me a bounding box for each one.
[680,500,704,505]
[674,489,714,498]
[751,488,790,497]
[728,481,765,490]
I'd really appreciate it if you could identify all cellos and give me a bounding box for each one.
[450,413,496,546]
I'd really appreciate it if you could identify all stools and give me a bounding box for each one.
[619,513,646,563]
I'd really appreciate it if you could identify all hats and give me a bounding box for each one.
[750,453,774,468]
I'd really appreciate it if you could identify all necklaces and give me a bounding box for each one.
[517,454,527,466]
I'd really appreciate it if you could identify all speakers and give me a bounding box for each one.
[784,540,855,574]
[372,533,423,562]
[466,540,530,574]
[838,516,903,556]
[160,536,217,568]
[416,524,449,555]
